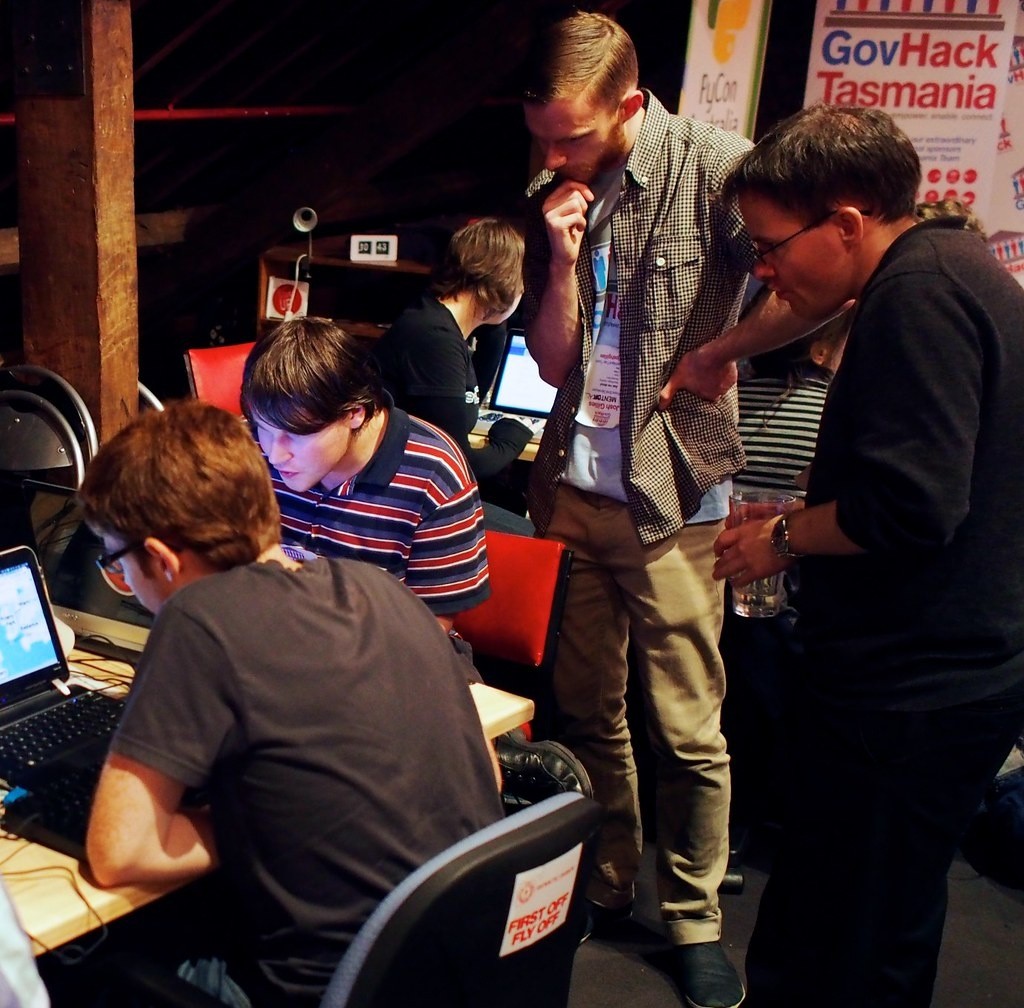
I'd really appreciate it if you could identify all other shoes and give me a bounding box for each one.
[573,896,634,945]
[677,941,747,1008]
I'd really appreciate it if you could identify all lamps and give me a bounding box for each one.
[294,208,318,257]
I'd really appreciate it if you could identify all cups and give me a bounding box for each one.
[729,493,796,618]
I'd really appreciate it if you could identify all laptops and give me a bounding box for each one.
[23,478,159,665]
[0,546,213,858]
[471,327,561,445]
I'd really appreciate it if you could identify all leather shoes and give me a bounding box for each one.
[491,726,594,811]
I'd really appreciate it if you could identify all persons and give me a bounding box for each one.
[710,106,1024,1008]
[80,218,526,1008]
[516,8,855,1008]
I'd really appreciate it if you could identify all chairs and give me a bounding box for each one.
[451,532,565,741]
[323,791,605,1007]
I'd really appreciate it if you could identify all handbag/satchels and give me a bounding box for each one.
[960,734,1024,891]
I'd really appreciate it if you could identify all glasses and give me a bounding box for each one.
[95,536,185,597]
[749,209,874,266]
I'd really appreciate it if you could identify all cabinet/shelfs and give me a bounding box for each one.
[256,239,433,337]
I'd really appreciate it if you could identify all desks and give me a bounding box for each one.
[0,607,535,956]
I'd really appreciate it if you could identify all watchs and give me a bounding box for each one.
[770,508,807,561]
